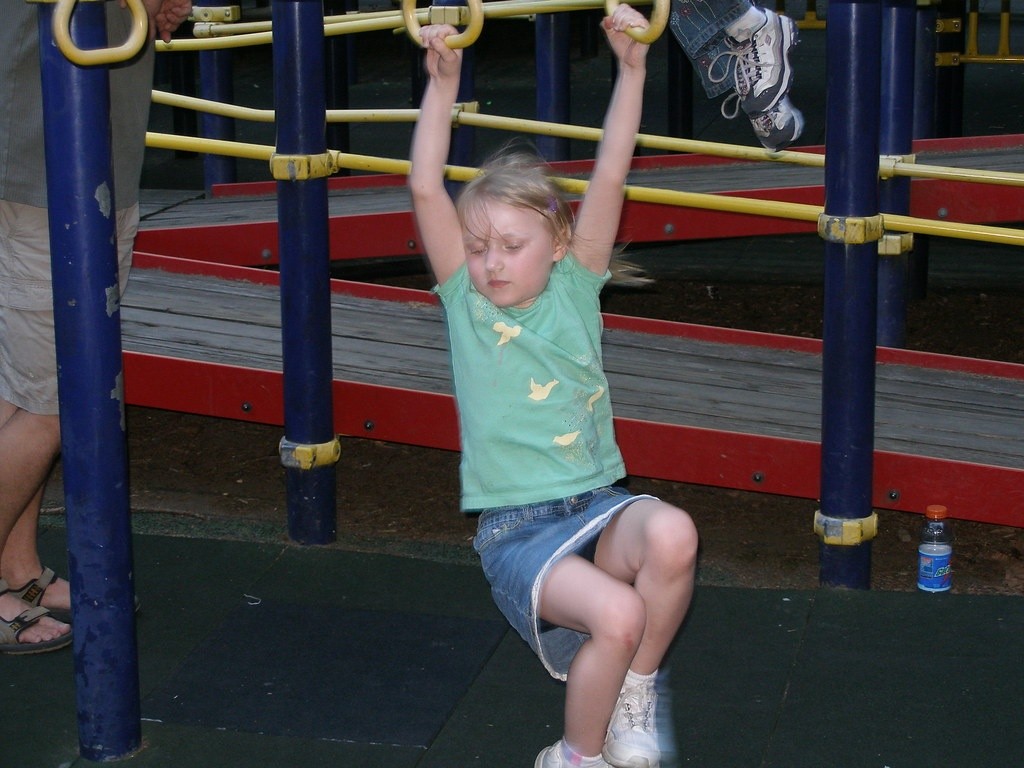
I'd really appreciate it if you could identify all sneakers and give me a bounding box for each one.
[707,7,801,119]
[602,679,661,768]
[722,92,803,151]
[534,739,615,768]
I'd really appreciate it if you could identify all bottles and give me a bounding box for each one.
[917,505,953,603]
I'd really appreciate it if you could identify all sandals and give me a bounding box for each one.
[8,561,145,623]
[0,577,72,652]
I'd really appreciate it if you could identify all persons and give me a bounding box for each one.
[408,3,699,768]
[668,0,805,160]
[0,0,196,659]
